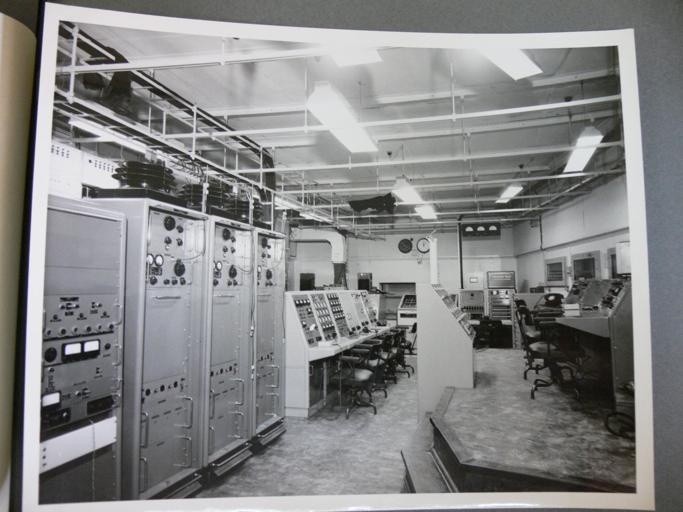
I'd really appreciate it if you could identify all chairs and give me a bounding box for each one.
[514,294,584,399]
[333,325,413,419]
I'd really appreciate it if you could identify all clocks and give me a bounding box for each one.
[416,238,429,253]
